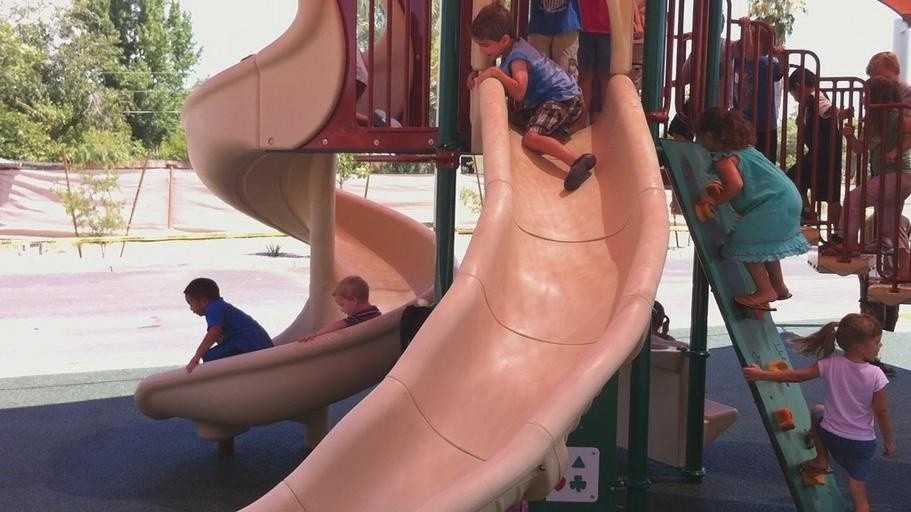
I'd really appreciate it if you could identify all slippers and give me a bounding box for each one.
[822,243,861,256]
[735,293,792,310]
[801,459,836,475]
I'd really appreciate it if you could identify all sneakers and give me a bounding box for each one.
[564,154,595,192]
[868,361,896,376]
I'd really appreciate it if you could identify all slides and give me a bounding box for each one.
[134,0,458,438]
[231,74,670,510]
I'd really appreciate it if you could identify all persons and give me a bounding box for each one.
[353,40,403,129]
[783,67,855,230]
[577,1,642,117]
[181,276,275,370]
[858,203,910,378]
[863,49,910,120]
[293,273,383,344]
[730,18,788,169]
[739,310,895,512]
[814,77,911,255]
[465,0,598,193]
[667,9,752,142]
[529,0,582,84]
[692,107,813,313]
